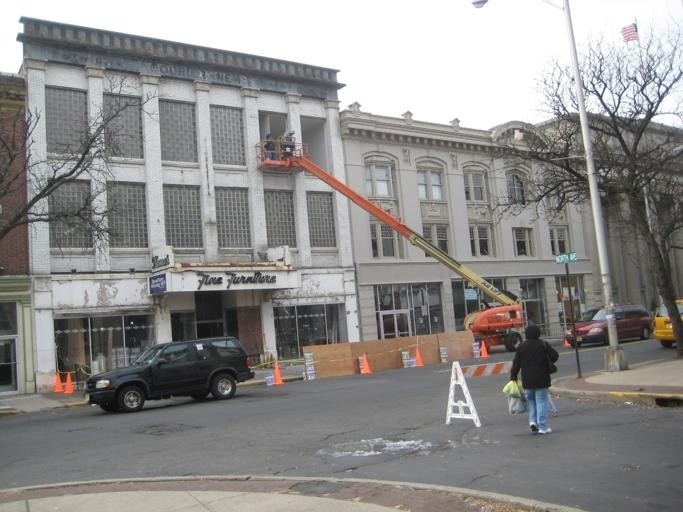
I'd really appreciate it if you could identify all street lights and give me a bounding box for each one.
[472,0,629,372]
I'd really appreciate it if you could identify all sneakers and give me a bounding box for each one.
[539,427,552,434]
[528,422,537,432]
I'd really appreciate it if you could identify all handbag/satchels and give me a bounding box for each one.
[540,341,557,374]
[508,394,527,415]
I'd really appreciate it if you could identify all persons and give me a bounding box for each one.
[281,130,295,160]
[509,323,560,435]
[262,132,275,160]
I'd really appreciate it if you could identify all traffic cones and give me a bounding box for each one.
[274,362,284,384]
[54,373,74,395]
[481,339,489,357]
[361,353,371,374]
[415,349,424,367]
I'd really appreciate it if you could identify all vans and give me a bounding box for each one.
[564,299,682,349]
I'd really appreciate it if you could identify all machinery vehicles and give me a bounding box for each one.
[256,136,526,352]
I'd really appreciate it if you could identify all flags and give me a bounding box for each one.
[620,23,640,44]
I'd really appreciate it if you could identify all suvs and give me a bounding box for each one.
[85,336,256,412]
